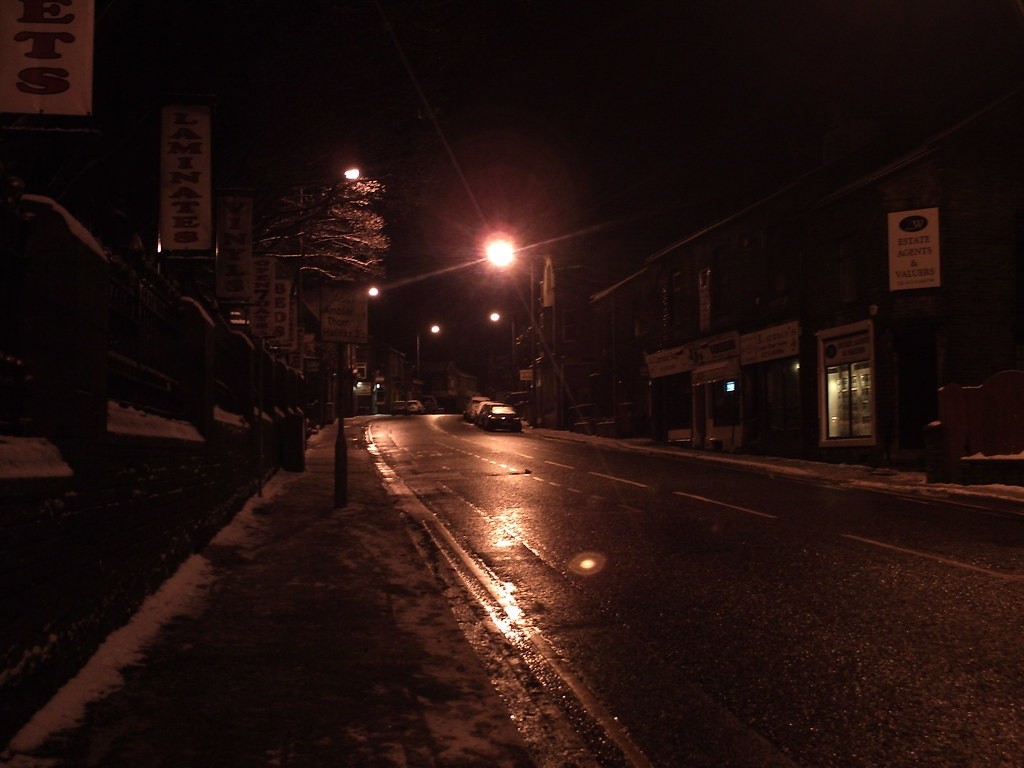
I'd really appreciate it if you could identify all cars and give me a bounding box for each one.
[465,396,522,433]
[391,400,425,416]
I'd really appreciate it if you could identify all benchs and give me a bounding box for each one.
[923,370,1024,486]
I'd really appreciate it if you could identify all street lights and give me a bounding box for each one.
[483,232,543,428]
